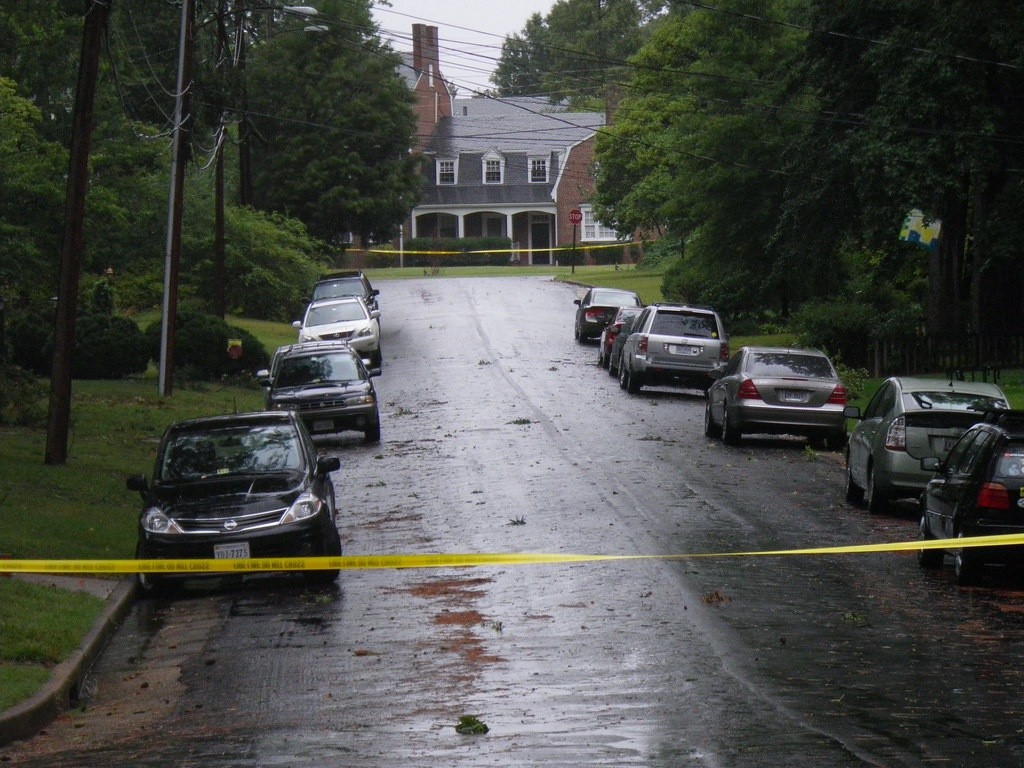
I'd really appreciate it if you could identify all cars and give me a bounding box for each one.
[705,346,847,446]
[260,343,381,441]
[257,340,371,406]
[292,294,382,368]
[917,410,1024,582]
[126,409,343,589]
[598,304,648,367]
[842,376,1011,510]
[573,287,648,343]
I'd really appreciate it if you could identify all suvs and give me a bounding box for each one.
[609,302,729,393]
[311,270,380,311]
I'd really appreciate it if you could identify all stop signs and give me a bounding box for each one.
[568,210,582,224]
